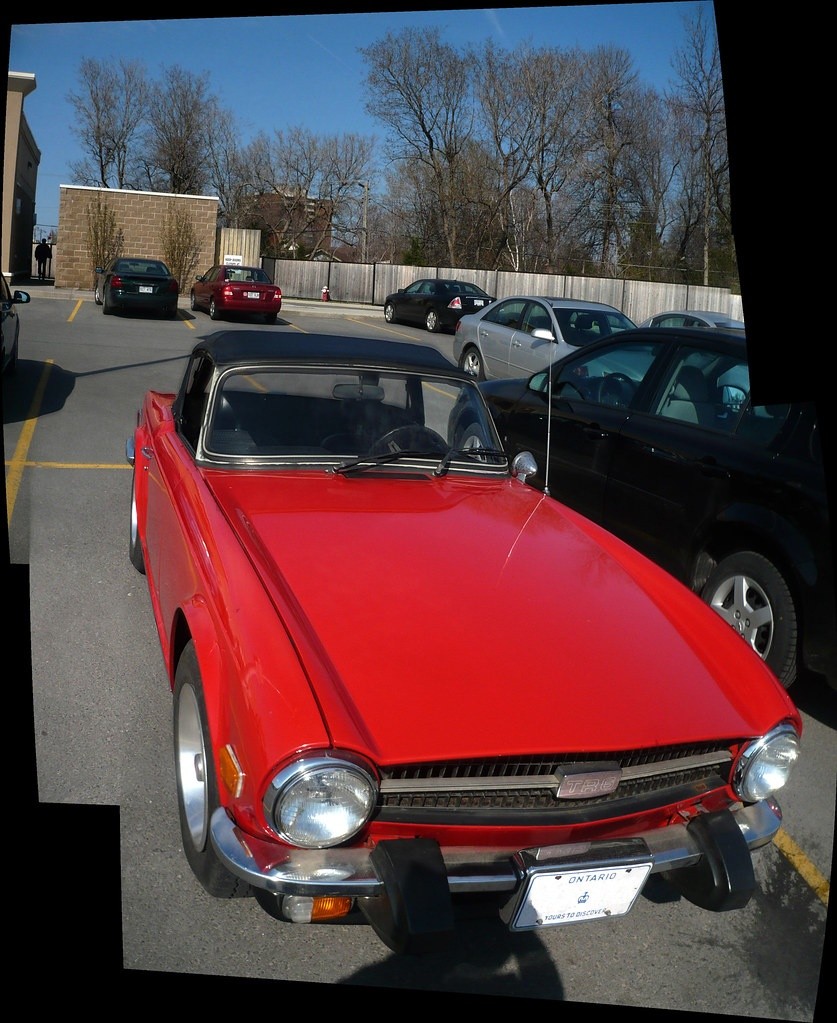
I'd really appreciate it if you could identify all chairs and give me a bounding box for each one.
[449,286,460,292]
[338,398,388,444]
[662,365,715,425]
[210,391,282,456]
[574,315,593,331]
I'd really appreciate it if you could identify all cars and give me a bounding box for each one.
[189,264,282,321]
[382,277,500,333]
[448,322,837,701]
[0,271,31,378]
[637,309,749,394]
[93,257,179,317]
[451,295,711,401]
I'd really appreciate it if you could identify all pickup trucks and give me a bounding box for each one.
[125,331,803,955]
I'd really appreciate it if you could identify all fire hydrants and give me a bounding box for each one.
[320,286,330,302]
